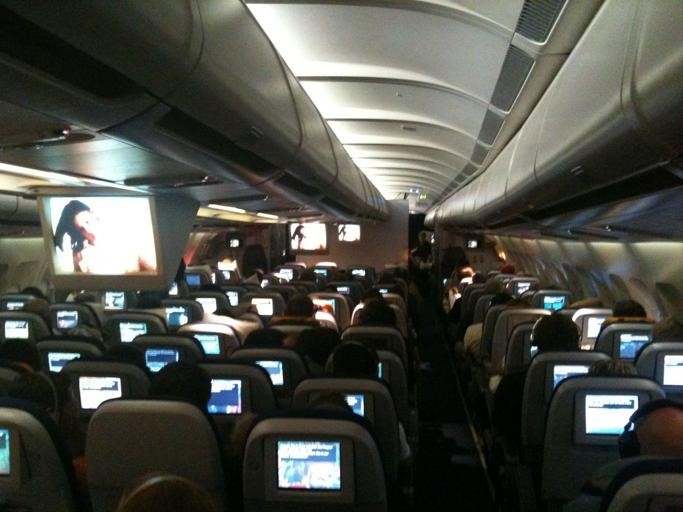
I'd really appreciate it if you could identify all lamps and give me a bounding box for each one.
[256,211,279,220]
[207,202,246,214]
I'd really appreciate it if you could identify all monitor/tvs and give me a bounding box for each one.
[581,316,611,347]
[165,306,192,327]
[56,311,80,330]
[145,348,180,373]
[120,322,148,343]
[654,353,683,392]
[207,379,247,420]
[288,223,328,253]
[5,321,31,340]
[337,225,361,243]
[74,376,132,417]
[1,428,16,479]
[253,360,290,388]
[500,279,568,365]
[169,269,388,319]
[194,333,222,356]
[48,352,84,374]
[104,291,126,311]
[38,193,168,293]
[545,361,597,406]
[575,390,651,447]
[274,437,346,495]
[7,301,25,312]
[312,391,373,424]
[614,331,654,365]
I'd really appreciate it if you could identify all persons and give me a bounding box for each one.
[412,232,432,264]
[1,257,682,511]
[290,225,324,250]
[52,200,151,275]
[338,226,347,242]
